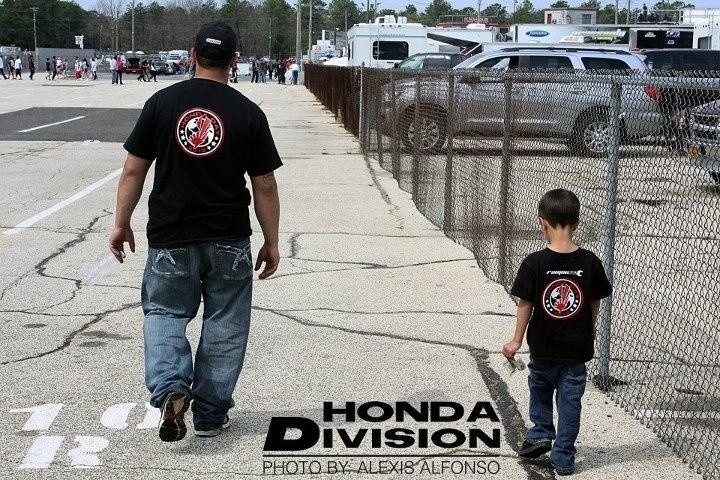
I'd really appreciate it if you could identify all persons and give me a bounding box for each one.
[0,54,301,86]
[501,188,613,476]
[106,20,285,443]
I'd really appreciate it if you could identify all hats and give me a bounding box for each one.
[194,20,237,60]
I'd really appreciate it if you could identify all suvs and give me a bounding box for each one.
[372,24,720,185]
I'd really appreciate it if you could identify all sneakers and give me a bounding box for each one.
[518,440,552,459]
[194,414,231,437]
[157,392,190,442]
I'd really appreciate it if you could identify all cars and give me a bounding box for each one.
[97,49,189,76]
